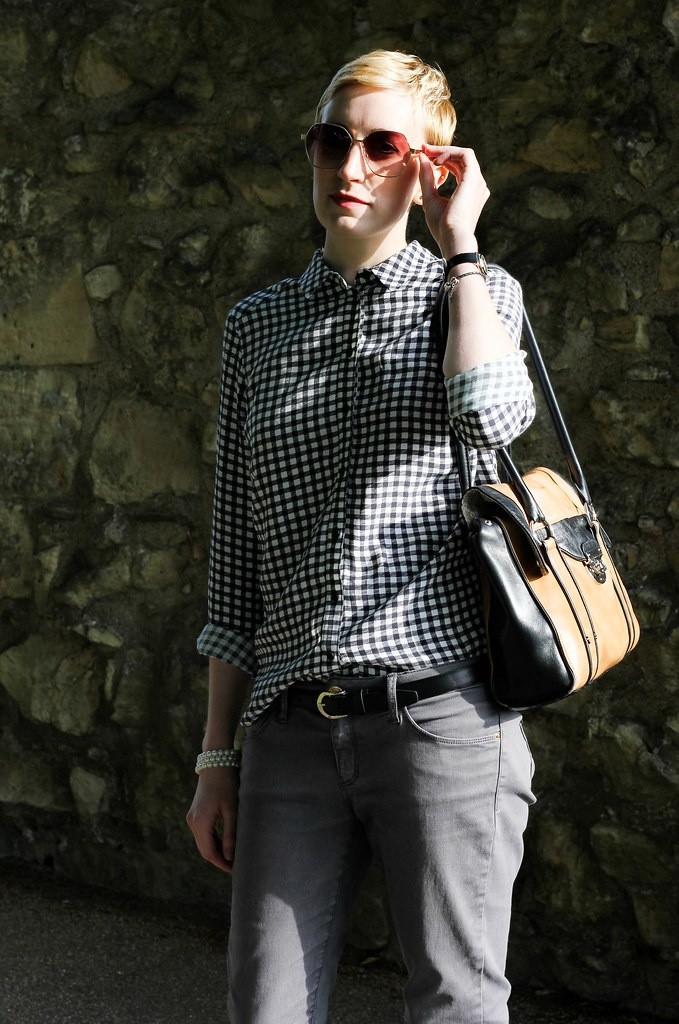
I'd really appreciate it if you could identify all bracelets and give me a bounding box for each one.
[444,271,486,304]
[194,749,238,775]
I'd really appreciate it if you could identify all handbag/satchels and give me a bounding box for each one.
[434,274,639,710]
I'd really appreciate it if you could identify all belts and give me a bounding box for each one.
[272,656,497,716]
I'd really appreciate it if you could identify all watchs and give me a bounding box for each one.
[444,250,488,283]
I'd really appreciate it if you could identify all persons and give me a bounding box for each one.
[182,50,537,1024]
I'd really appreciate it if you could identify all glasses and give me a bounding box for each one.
[301,123,423,178]
